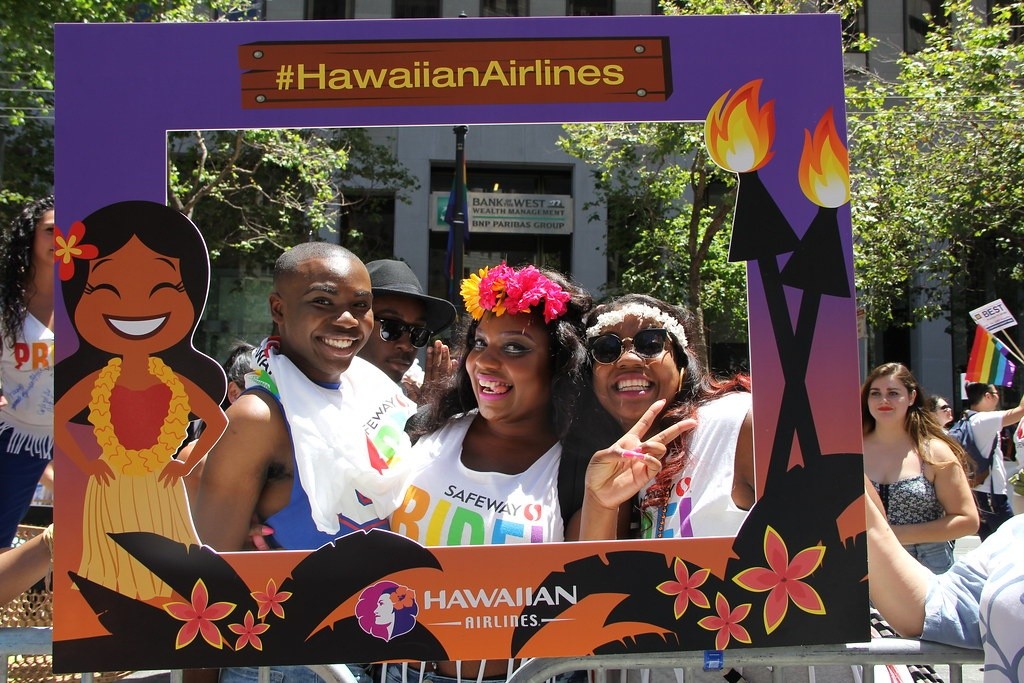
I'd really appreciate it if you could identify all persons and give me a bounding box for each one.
[357,259,460,409]
[865,487,1024,683]
[861,363,980,575]
[0,193,54,607]
[962,380,1024,543]
[176,340,274,551]
[557,292,756,541]
[194,242,420,552]
[923,394,953,434]
[389,266,699,547]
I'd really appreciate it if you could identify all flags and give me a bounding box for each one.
[965,324,1021,387]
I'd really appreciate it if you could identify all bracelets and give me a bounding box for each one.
[43,523,53,594]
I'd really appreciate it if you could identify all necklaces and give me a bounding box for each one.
[658,479,672,538]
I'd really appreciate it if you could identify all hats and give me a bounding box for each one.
[365,259,456,338]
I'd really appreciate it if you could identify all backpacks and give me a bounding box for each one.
[948,412,998,489]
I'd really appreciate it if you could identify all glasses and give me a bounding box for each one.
[990,390,1000,396]
[586,328,678,365]
[374,315,435,349]
[941,405,951,409]
